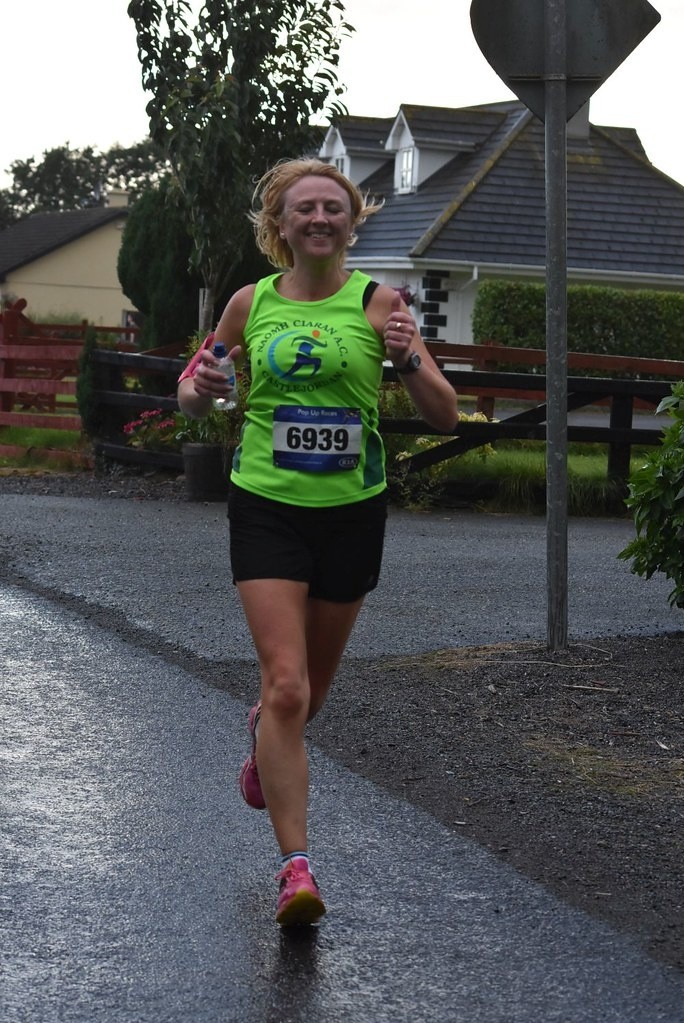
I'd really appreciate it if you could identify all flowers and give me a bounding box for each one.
[124,409,177,447]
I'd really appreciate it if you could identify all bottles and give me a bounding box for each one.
[207,343,240,411]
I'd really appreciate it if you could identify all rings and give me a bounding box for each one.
[395,320,402,332]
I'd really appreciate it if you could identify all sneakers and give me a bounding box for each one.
[274,851,326,926]
[239,701,267,809]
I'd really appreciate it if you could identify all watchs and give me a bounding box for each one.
[392,351,422,375]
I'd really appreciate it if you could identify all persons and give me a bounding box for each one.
[175,160,459,934]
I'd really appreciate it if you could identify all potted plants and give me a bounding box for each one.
[168,403,231,500]
